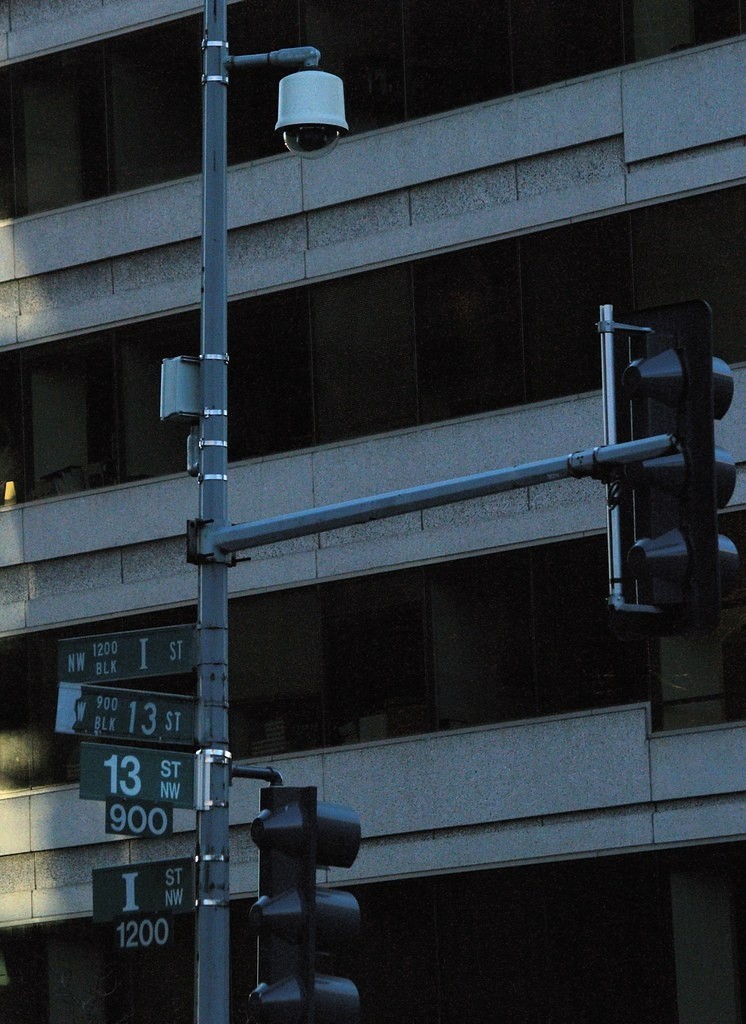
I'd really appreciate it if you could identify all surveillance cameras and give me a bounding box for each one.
[274,71,349,158]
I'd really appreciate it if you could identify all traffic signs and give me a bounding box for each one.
[54,684,197,749]
[76,743,196,810]
[89,860,196,924]
[54,623,196,684]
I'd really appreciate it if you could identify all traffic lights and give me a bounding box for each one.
[252,782,363,1024]
[630,296,737,634]
